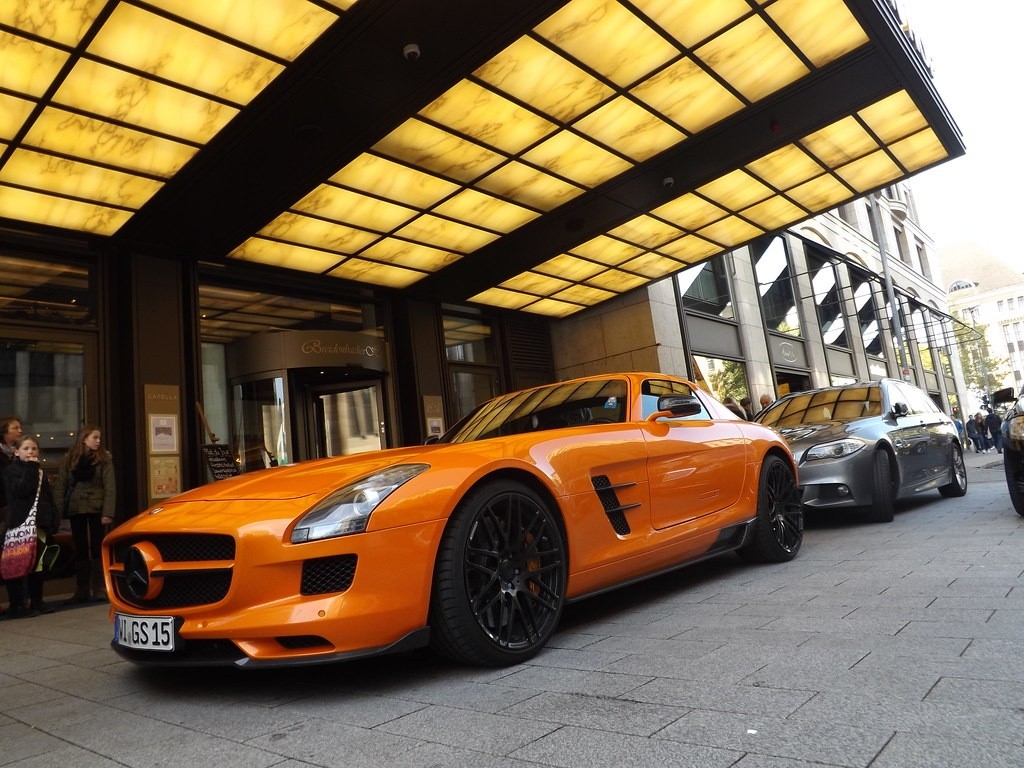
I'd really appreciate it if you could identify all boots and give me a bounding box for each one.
[64,582,90,604]
[92,578,108,601]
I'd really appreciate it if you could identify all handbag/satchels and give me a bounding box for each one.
[1,501,37,579]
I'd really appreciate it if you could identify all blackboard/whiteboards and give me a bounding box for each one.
[202,443,241,481]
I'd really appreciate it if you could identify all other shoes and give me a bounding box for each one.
[8,604,27,619]
[29,601,53,617]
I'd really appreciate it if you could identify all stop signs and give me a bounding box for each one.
[903,370,911,376]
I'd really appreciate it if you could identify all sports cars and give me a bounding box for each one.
[100,371,805,672]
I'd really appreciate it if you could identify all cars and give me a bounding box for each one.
[749,376,968,524]
[991,382,1024,517]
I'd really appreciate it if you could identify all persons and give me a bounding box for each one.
[722,393,773,421]
[53,426,115,605]
[950,408,1003,454]
[0,417,60,617]
